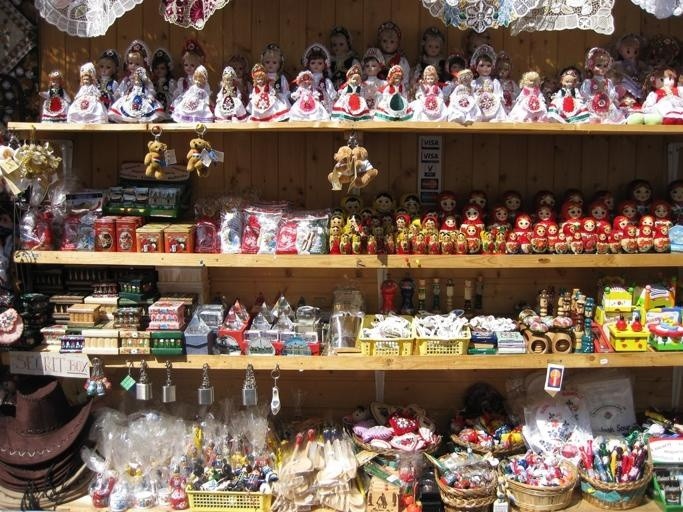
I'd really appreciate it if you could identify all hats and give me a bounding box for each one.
[0,380,96,511]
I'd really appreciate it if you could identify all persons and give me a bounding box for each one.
[37,20,682,124]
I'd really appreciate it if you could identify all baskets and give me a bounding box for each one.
[449,409,527,458]
[498,453,579,511]
[352,429,652,512]
[359,314,472,355]
[350,427,442,476]
[434,453,497,512]
[578,457,653,510]
[184,484,272,512]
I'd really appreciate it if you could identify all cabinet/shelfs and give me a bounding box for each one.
[7,0,682,512]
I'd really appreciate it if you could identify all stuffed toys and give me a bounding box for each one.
[326,146,354,190]
[143,136,167,179]
[185,138,214,177]
[350,147,377,189]
